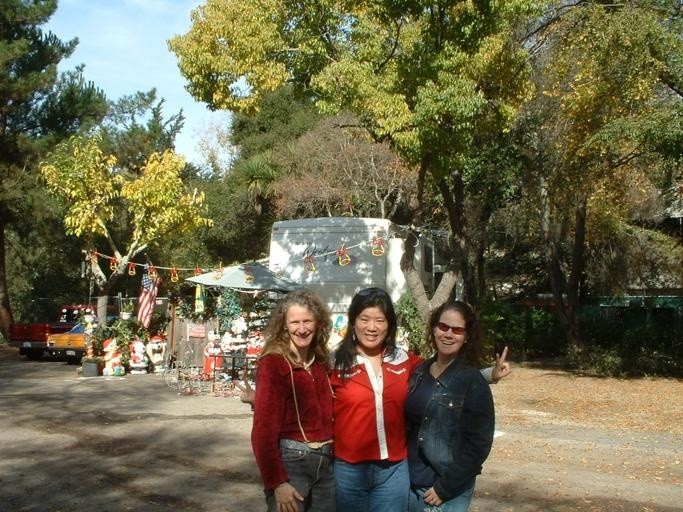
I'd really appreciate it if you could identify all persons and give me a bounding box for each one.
[251,288,337,512]
[233,288,512,512]
[404,302,495,512]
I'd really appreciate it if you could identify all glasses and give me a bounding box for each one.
[438,322,466,334]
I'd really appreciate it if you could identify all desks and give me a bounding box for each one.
[209,354,258,392]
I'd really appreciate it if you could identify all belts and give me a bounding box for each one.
[280,439,332,454]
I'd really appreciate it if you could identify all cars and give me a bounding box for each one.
[9,304,118,365]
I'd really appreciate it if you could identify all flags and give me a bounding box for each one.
[138,273,162,330]
